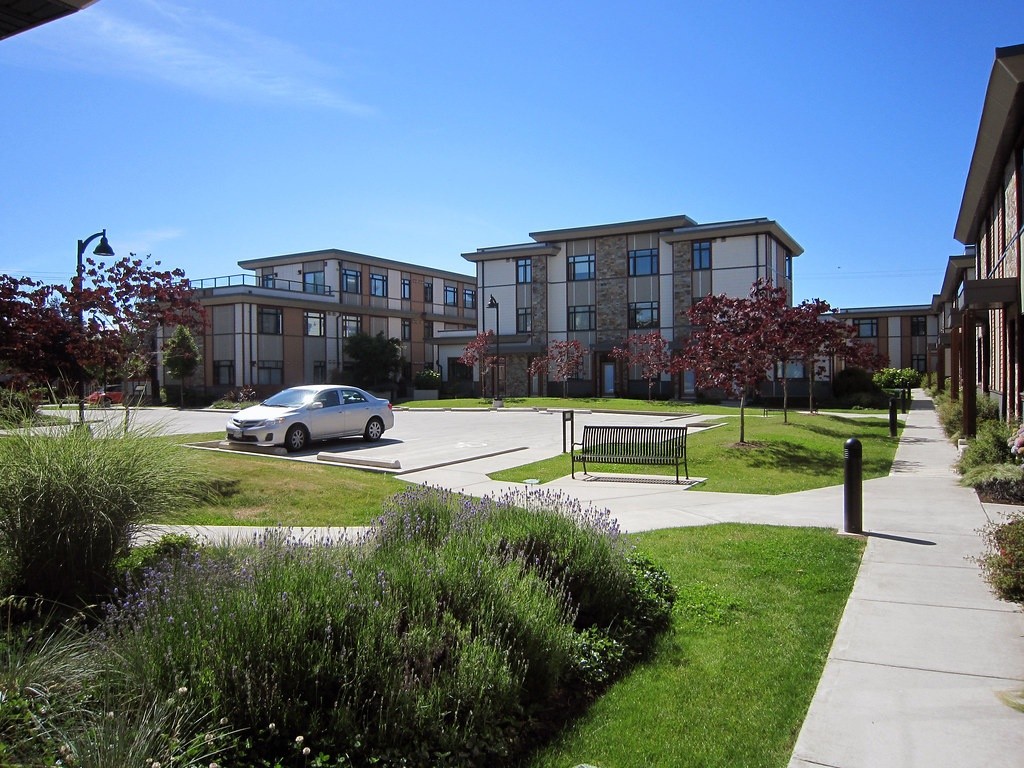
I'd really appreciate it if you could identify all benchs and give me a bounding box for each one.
[343,393,365,404]
[571,424,689,485]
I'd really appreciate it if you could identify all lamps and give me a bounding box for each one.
[324,262,327,266]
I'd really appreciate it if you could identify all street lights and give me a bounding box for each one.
[88,313,110,407]
[485,293,503,408]
[71,228,115,438]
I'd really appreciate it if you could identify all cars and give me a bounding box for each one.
[225,385,394,453]
[84,384,122,406]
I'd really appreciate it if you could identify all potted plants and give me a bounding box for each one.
[413,369,440,400]
